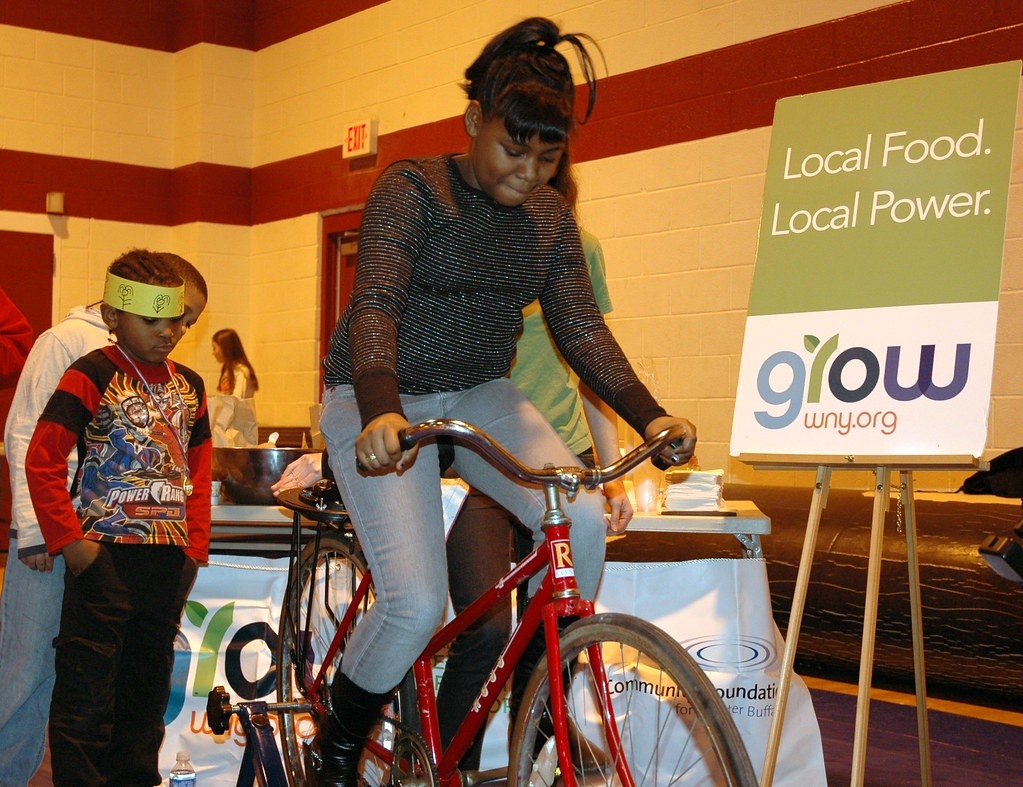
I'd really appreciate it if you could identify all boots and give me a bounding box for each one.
[508,596,610,772]
[303,662,393,787]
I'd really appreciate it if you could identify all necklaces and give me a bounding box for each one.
[108,339,195,496]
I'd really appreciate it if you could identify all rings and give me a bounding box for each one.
[671,454,680,464]
[367,453,376,463]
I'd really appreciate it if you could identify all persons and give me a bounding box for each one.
[24,249,214,787]
[1,252,208,787]
[303,15,697,787]
[210,328,259,448]
[446,220,635,773]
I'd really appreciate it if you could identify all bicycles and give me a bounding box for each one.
[277,419,763,786]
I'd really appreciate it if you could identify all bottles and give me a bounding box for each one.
[169,752,197,787]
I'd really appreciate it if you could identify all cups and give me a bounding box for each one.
[633,458,664,513]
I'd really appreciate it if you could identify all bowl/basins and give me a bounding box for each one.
[211,447,306,506]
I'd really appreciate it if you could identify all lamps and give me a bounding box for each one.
[979,522,1023,584]
